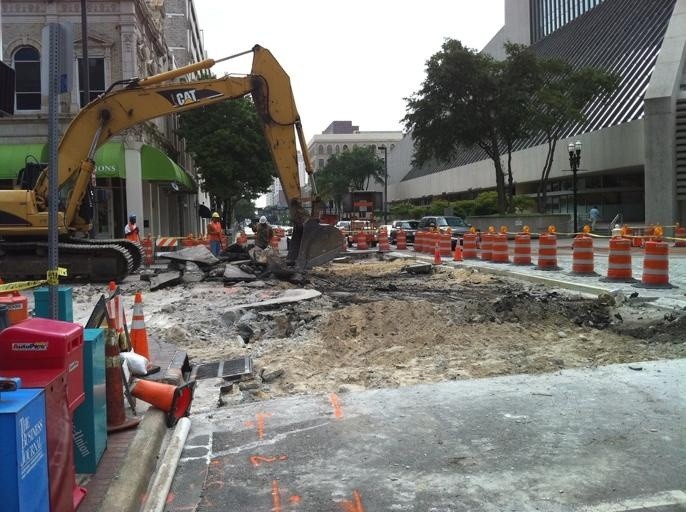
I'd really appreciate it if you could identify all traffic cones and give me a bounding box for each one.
[453,236,463,261]
[106,331,139,432]
[131,291,161,377]
[106,282,126,330]
[433,240,443,266]
[127,377,195,427]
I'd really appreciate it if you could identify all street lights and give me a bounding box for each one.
[568,142,582,232]
[378,147,387,224]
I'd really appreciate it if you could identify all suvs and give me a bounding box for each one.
[419,215,481,250]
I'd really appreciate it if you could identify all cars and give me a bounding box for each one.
[335,220,420,244]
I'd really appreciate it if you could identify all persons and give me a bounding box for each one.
[251,215,273,250]
[206,212,224,259]
[589,206,600,231]
[124,214,143,246]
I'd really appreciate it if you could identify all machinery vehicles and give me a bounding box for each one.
[0,45,347,284]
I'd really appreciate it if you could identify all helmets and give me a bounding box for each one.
[259,216,267,223]
[212,212,220,217]
[130,215,136,219]
